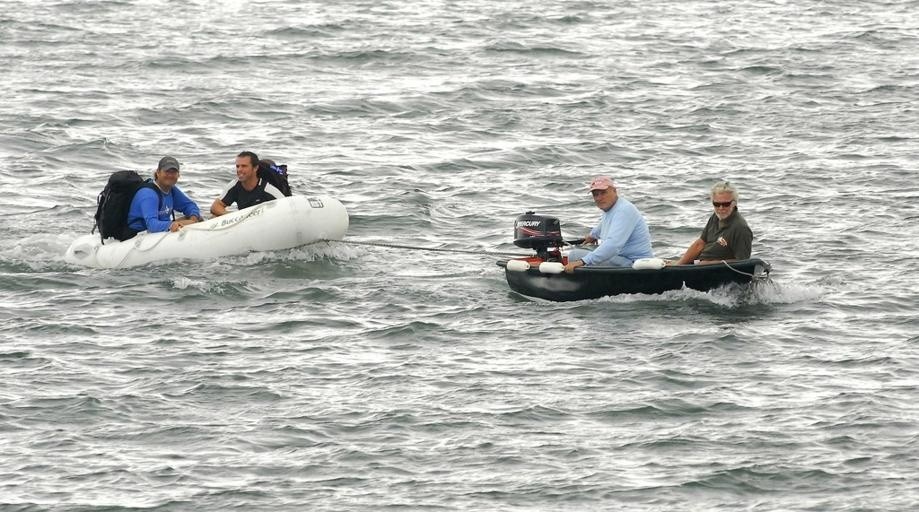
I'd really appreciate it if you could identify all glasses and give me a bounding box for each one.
[712,198,735,208]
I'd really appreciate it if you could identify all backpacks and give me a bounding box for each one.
[258,159,292,198]
[92,170,163,242]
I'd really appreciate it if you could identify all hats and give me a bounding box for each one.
[158,157,180,173]
[587,175,616,197]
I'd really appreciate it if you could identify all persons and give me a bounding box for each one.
[208,149,295,219]
[560,174,653,274]
[120,156,202,245]
[664,180,752,270]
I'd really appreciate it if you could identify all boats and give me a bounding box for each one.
[63,194,350,276]
[497,256,772,303]
